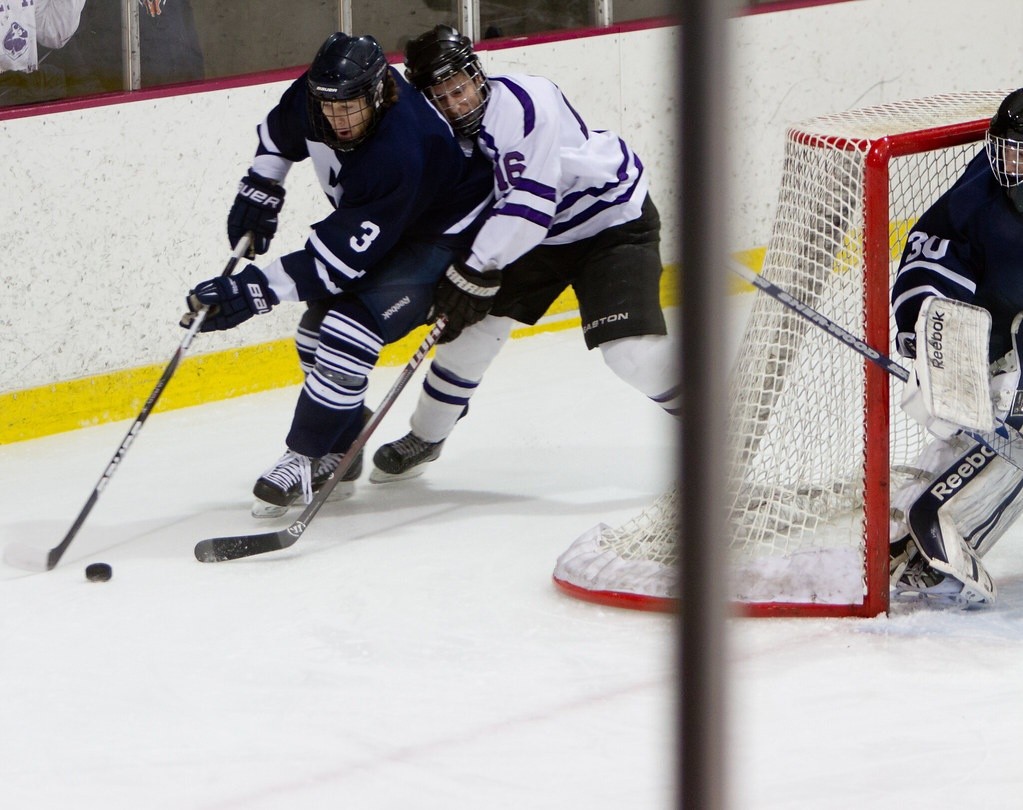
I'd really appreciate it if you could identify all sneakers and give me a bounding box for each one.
[312,405,373,502]
[370,403,468,485]
[251,447,321,519]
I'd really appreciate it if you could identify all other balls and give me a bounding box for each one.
[85,562,113,583]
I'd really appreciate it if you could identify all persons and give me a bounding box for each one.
[887,88,1023,608]
[368,24,681,484]
[0,0,205,105]
[179,31,496,520]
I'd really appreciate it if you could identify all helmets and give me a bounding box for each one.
[403,23,492,141]
[986,88,1023,213]
[304,32,390,152]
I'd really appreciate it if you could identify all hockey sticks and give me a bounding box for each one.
[194,311,450,563]
[718,256,1023,473]
[0,229,255,572]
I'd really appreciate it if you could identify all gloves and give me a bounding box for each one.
[426,261,502,345]
[227,169,286,261]
[179,264,280,333]
